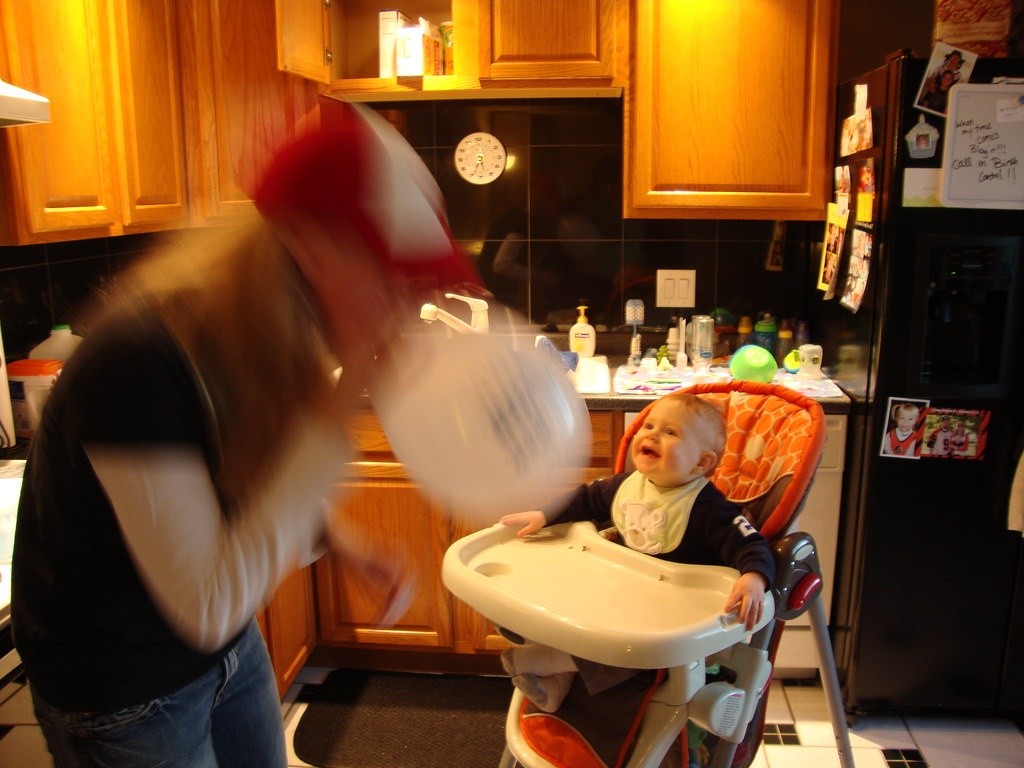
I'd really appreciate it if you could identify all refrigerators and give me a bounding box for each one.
[832,48,1024,722]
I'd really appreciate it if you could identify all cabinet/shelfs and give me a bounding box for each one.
[316,398,623,674]
[275,0,625,104]
[0,0,319,245]
[623,0,839,218]
[258,561,316,699]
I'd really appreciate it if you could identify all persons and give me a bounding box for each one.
[10,101,484,768]
[883,403,970,458]
[909,49,967,152]
[499,393,772,714]
[812,119,874,306]
[486,188,593,303]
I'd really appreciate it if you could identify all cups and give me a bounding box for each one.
[439,20,456,76]
[798,342,823,379]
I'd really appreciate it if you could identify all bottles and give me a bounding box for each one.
[28,324,85,360]
[735,313,807,368]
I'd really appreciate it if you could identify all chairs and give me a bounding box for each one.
[441,381,856,768]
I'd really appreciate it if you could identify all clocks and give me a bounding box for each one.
[454,132,506,186]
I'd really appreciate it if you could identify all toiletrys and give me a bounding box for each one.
[570,307,596,355]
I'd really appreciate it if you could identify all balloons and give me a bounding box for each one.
[370,293,589,523]
